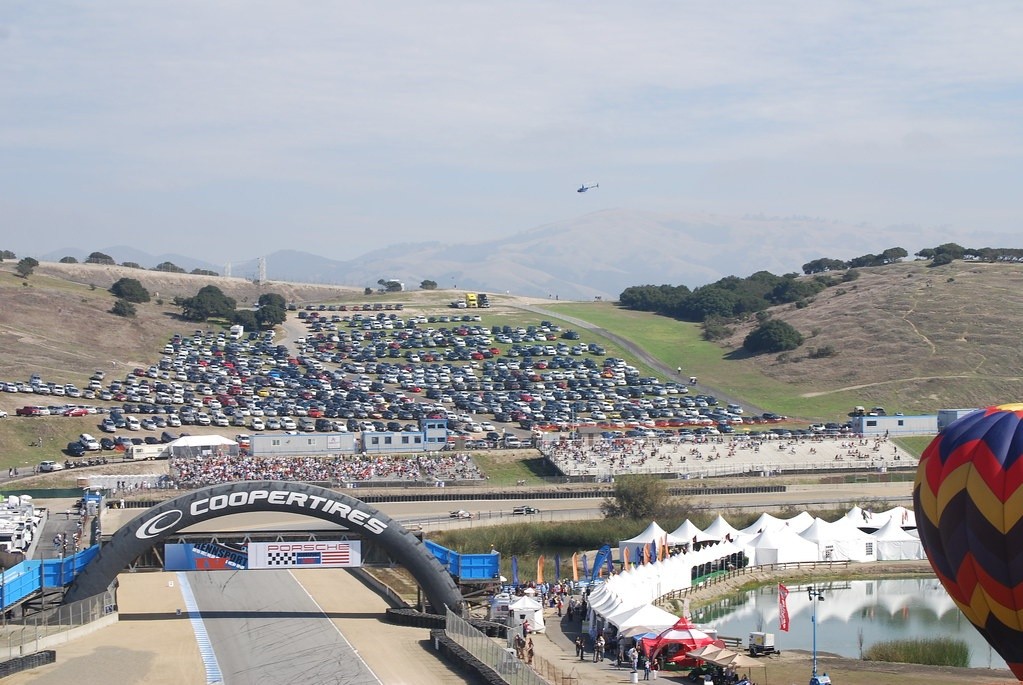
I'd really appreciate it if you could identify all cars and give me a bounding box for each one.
[2,302,854,474]
[853,406,887,416]
[456,300,467,308]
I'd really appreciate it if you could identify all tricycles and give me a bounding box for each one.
[689,377,698,387]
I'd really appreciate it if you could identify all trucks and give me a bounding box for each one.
[490,593,520,624]
[1,493,41,554]
[467,293,489,308]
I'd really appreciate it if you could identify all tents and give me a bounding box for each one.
[585,504,929,669]
[524,587,536,597]
[508,596,546,634]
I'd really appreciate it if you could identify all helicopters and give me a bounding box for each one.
[577,183,600,193]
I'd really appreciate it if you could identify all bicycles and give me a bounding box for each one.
[677,366,682,375]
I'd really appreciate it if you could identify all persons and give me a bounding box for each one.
[575,624,660,680]
[508,578,571,617]
[701,661,749,685]
[112,497,124,508]
[567,596,587,621]
[543,438,599,475]
[834,432,900,465]
[779,435,824,456]
[523,619,530,638]
[690,435,766,461]
[591,438,686,472]
[52,530,79,559]
[176,450,481,487]
[116,478,174,491]
[514,633,535,665]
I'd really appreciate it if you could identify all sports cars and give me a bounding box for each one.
[515,506,540,516]
[448,509,474,519]
